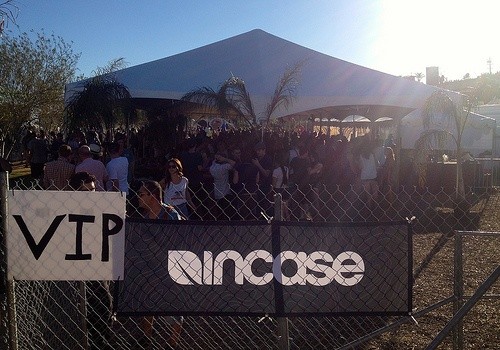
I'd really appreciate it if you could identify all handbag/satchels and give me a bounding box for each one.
[253,175,273,205]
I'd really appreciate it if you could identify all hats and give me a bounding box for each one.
[89,144,101,152]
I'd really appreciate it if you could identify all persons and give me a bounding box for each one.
[64,174,112,349]
[137,180,183,350]
[22,123,498,225]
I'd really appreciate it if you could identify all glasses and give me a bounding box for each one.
[168,165,177,169]
[137,191,152,198]
